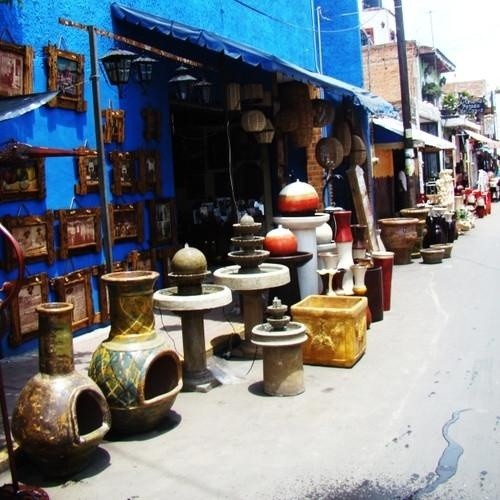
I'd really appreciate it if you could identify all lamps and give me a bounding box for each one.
[98,44,212,106]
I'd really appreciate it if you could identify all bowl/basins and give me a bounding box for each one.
[420,248,445,263]
[430,244,454,258]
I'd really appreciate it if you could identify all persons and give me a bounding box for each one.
[494,155,500,204]
[477,167,490,192]
